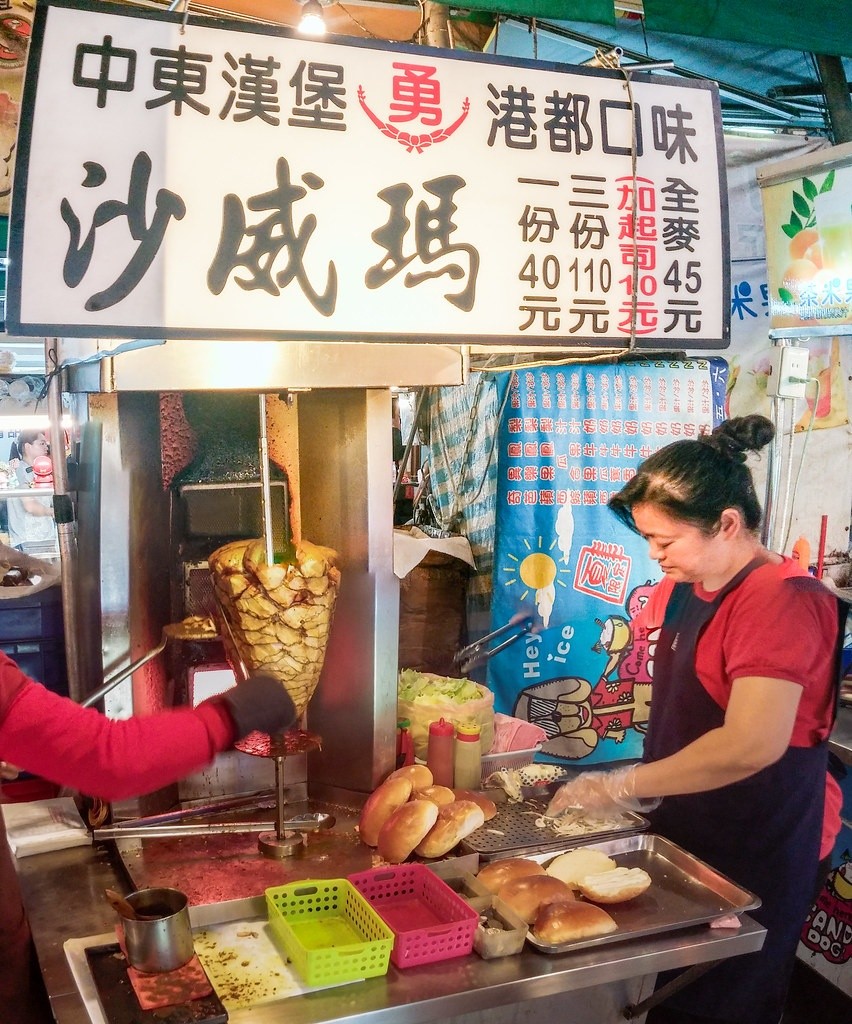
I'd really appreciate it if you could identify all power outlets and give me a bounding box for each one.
[766,346,809,399]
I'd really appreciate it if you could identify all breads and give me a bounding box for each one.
[475,849,652,947]
[359,764,498,862]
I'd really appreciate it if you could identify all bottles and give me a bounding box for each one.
[429,717,454,788]
[397,717,415,768]
[455,723,482,790]
[792,536,810,573]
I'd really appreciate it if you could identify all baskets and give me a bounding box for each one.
[264,875,395,990]
[347,862,479,968]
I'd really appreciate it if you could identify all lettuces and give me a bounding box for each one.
[395,669,495,759]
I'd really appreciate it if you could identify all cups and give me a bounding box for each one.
[806,369,830,419]
[119,888,195,973]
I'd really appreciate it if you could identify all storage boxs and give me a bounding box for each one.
[264,878,396,987]
[180,481,284,537]
[434,866,529,959]
[347,863,480,971]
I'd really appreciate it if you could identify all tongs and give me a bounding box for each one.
[94,785,336,839]
[454,612,536,672]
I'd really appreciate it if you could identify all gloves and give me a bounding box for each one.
[215,673,298,739]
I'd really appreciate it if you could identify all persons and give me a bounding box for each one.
[4,429,62,571]
[545,411,848,1024]
[0,650,295,1024]
